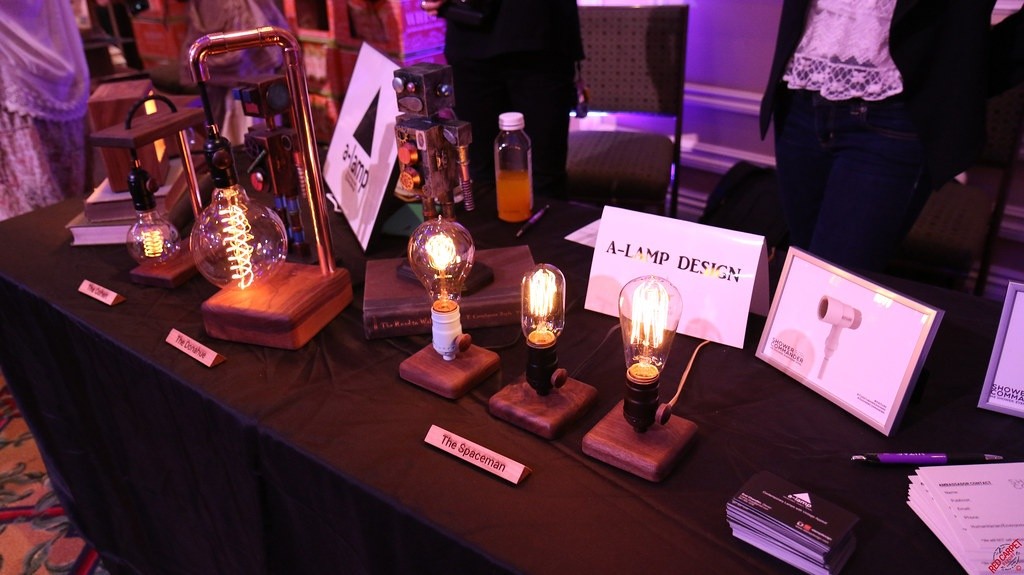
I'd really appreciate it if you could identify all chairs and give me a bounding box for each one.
[878,85,1024,294]
[570,6,693,221]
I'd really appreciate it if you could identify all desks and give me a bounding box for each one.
[1,169,1024,575]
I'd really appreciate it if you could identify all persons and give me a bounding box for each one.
[422,0,589,201]
[0,0,91,218]
[182,0,290,148]
[96,0,146,72]
[759,0,998,276]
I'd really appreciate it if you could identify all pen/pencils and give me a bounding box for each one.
[516,204,551,238]
[851,452,1004,465]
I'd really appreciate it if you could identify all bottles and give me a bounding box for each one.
[495,113,534,223]
